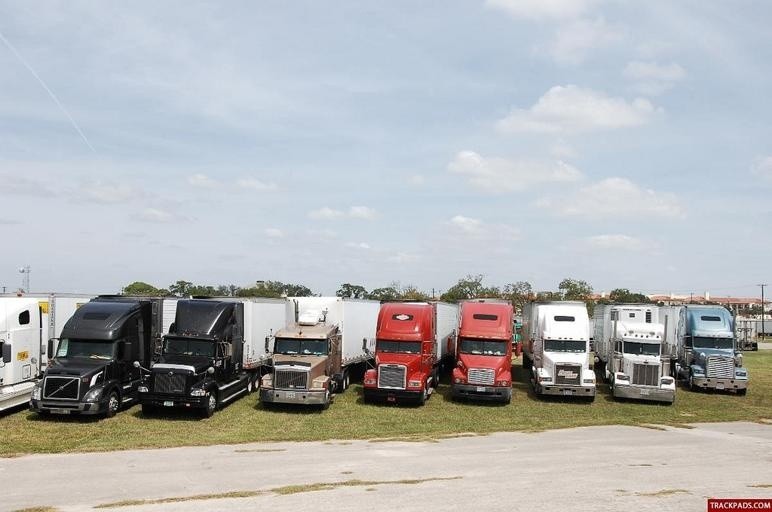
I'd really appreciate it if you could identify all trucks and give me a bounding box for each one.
[522,299,749,404]
